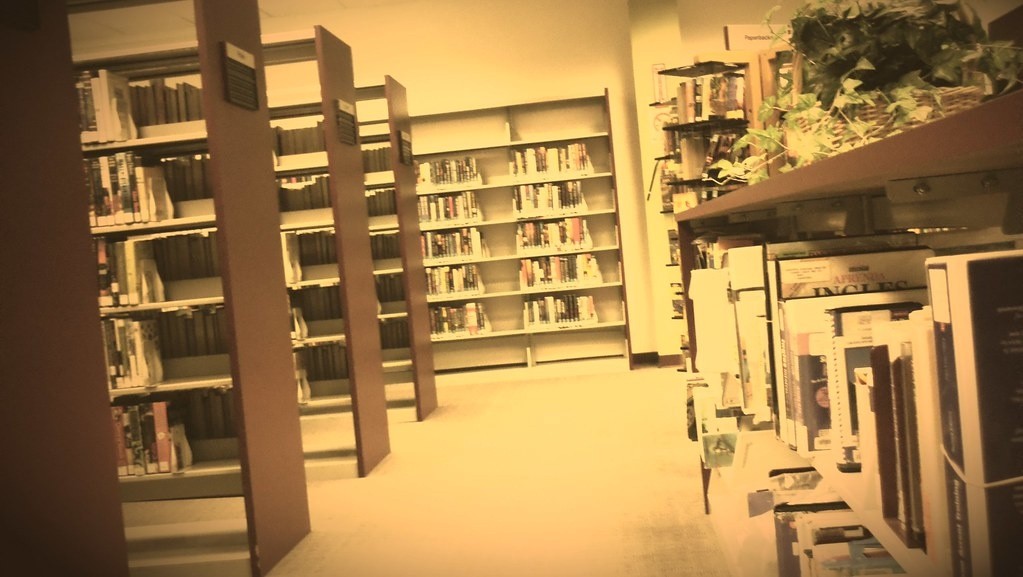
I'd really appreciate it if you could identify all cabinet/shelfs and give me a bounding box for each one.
[405,88,633,372]
[649,46,1023,577]
[0,0,439,577]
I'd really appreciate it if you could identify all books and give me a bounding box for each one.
[508,144,602,330]
[73,67,411,480]
[413,157,492,341]
[656,55,1023,577]
